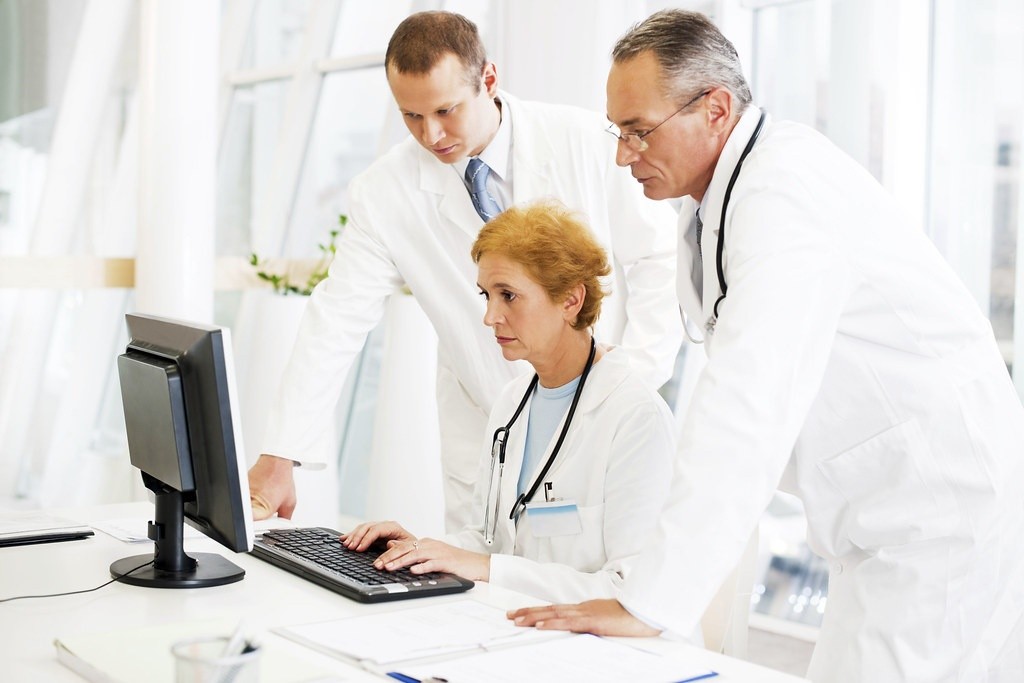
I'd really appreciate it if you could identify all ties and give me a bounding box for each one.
[695,209,703,262]
[465,158,501,222]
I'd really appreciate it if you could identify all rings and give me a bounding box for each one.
[412,540,420,550]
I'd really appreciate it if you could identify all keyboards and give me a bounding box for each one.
[248,526,475,603]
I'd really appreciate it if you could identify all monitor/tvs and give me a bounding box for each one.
[111,314,254,587]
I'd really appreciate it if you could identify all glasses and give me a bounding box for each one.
[604,92,710,153]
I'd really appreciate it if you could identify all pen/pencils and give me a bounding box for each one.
[544,481,553,501]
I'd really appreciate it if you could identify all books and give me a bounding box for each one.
[266,598,719,682]
[50,622,394,683]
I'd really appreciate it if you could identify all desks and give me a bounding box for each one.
[0,503,806,683]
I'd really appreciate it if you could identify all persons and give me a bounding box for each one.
[506,6,1024,683]
[246,9,690,540]
[337,196,708,649]
